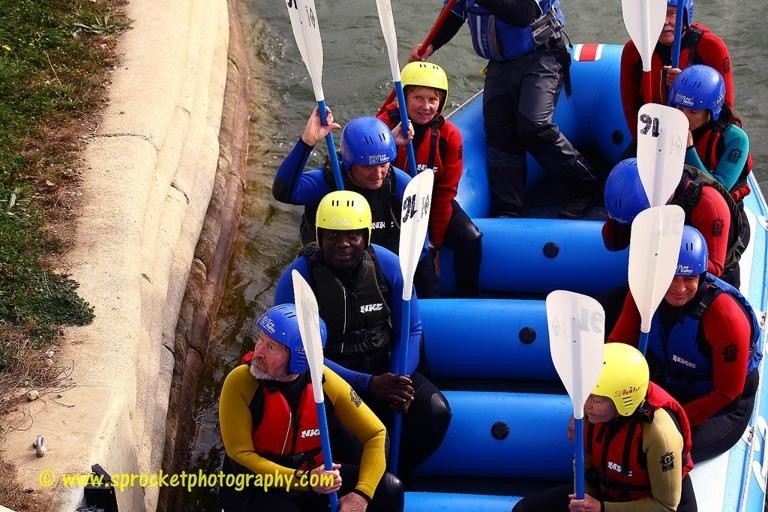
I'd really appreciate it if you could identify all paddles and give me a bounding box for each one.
[629,205,685,356]
[292,269,342,512]
[285,0,345,191]
[392,169,435,475]
[546,290,607,500]
[636,103,690,210]
[621,0,668,104]
[376,1,418,176]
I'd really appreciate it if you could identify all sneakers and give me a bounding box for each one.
[559,196,594,219]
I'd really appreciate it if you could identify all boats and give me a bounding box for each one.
[403,43,768,512]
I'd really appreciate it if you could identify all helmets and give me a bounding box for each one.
[674,225,709,276]
[315,190,372,247]
[256,303,327,375]
[590,342,650,416]
[668,1,694,26]
[340,115,397,173]
[400,61,448,117]
[668,65,725,121]
[604,157,651,224]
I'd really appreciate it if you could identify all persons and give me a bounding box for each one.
[602,158,750,291]
[377,61,483,299]
[408,0,601,219]
[620,0,734,161]
[667,63,753,208]
[512,342,697,512]
[273,104,428,298]
[607,224,763,464]
[274,190,451,492]
[219,303,404,512]
